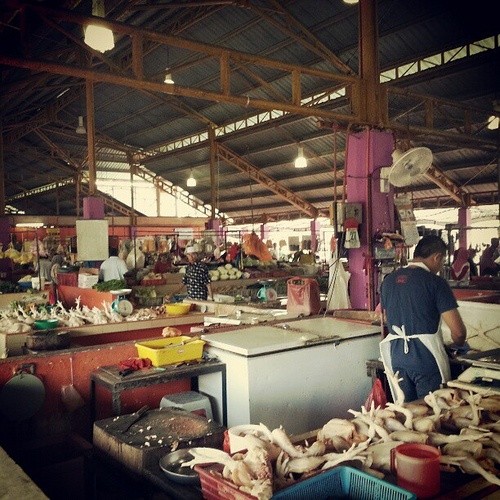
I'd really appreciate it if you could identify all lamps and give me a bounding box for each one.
[187,168,196,187]
[163,45,175,84]
[83,0,115,53]
[76,86,86,134]
[295,147,306,168]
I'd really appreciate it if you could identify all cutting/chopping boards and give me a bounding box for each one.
[26,330,71,350]
[93,407,227,475]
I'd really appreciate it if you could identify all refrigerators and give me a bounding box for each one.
[198,317,383,434]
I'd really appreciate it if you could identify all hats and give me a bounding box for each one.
[184,247,198,255]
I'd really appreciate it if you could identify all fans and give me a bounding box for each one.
[380,146,434,193]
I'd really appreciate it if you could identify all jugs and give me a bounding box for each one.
[390,444,441,497]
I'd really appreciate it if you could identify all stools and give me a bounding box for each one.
[160,391,213,421]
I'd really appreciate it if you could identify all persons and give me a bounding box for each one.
[35,253,65,280]
[450,238,500,282]
[170,241,181,256]
[154,253,178,273]
[229,242,247,267]
[100,247,134,282]
[180,239,228,263]
[379,235,467,402]
[164,246,215,302]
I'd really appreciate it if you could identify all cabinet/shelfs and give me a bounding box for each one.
[373,244,413,305]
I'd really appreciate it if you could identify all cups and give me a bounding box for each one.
[228,424,258,454]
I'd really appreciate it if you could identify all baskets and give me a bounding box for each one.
[267,466,416,500]
[193,449,324,500]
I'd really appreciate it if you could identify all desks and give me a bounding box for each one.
[90,358,227,443]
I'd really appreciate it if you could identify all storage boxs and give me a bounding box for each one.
[135,336,206,368]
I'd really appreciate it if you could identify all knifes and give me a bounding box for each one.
[117,405,150,432]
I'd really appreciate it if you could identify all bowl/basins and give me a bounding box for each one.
[163,303,192,315]
[34,319,59,329]
[159,447,199,484]
